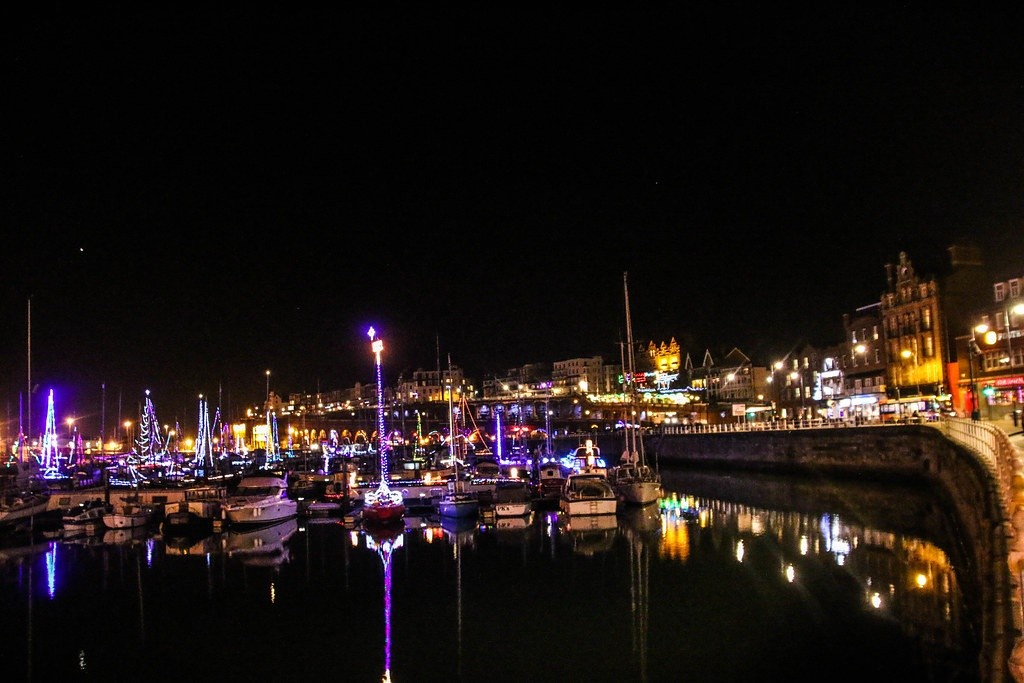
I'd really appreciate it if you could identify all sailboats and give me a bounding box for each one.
[608,269,661,505]
[0,299,612,523]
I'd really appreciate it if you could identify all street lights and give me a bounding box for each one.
[967,323,997,421]
[901,348,920,396]
[1004,302,1024,428]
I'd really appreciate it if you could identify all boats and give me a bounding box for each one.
[62,497,110,530]
[560,473,617,515]
[102,502,159,531]
[309,493,352,516]
[164,486,220,531]
[222,475,301,522]
